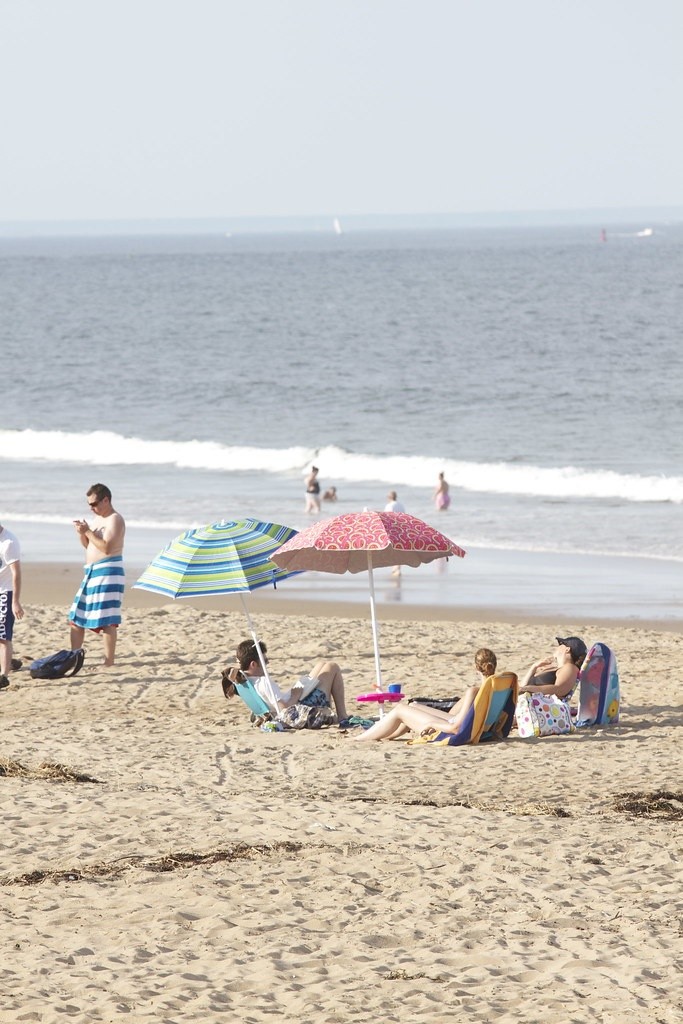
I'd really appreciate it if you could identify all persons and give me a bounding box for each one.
[237,639,363,729]
[357,648,498,745]
[303,464,450,513]
[0,521,26,690]
[520,637,586,696]
[68,482,125,672]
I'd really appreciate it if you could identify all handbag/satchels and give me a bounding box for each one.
[515,691,575,739]
[276,703,338,730]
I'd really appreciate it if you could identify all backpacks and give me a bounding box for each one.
[29,648,85,679]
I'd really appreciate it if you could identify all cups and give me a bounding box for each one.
[388,684,401,693]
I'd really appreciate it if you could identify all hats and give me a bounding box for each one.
[555,636,587,665]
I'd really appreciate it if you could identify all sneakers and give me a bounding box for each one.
[0,674,10,691]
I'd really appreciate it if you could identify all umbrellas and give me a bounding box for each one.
[268,508,466,721]
[131,519,301,717]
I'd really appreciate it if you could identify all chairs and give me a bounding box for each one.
[403,646,595,746]
[227,669,276,727]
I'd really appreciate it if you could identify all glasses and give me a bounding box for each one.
[89,496,105,506]
